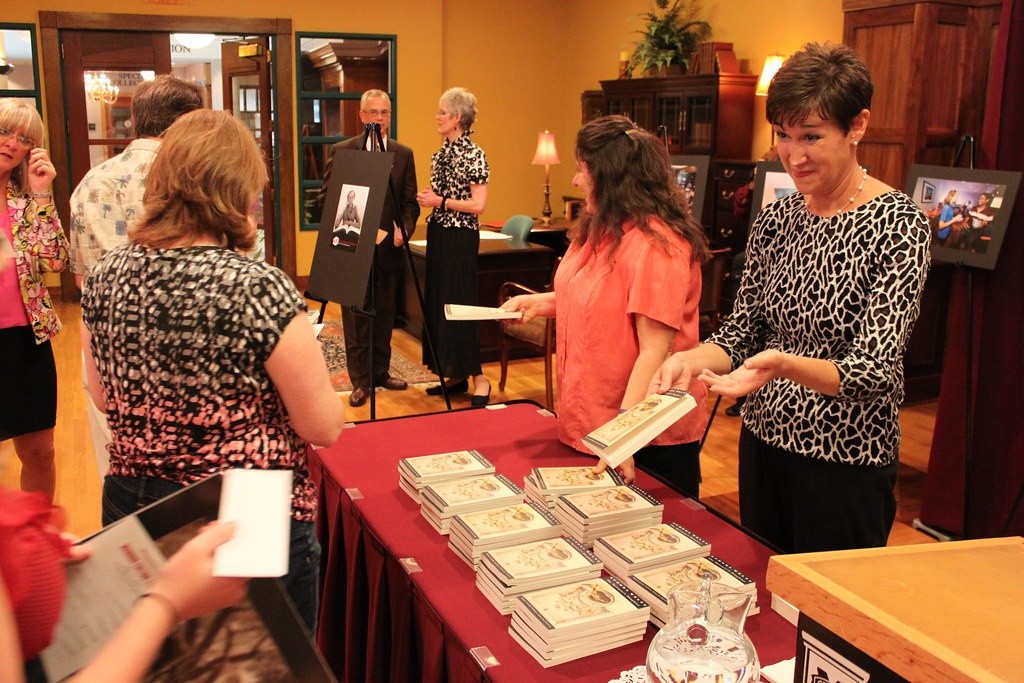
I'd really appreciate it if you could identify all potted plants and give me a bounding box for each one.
[627,0,711,76]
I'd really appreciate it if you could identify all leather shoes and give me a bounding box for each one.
[374,375,408,390]
[349,384,367,405]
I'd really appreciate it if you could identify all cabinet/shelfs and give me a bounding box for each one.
[843,0,1010,378]
[582,75,758,284]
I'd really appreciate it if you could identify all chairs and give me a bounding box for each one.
[496,257,565,411]
[501,215,534,240]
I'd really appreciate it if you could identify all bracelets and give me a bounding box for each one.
[34,192,52,198]
[133,592,180,636]
[440,197,449,211]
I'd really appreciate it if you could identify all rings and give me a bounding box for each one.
[45,161,50,166]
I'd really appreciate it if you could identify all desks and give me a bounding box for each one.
[300,399,797,683]
[397,224,554,363]
[483,217,580,254]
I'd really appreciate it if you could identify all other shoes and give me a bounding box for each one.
[425,376,468,395]
[471,375,491,406]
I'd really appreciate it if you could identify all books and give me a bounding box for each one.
[581,388,697,469]
[443,304,523,321]
[716,50,740,74]
[523,466,665,549]
[625,555,760,629]
[686,42,734,74]
[593,522,712,582]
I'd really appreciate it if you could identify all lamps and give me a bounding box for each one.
[530,132,561,226]
[754,54,787,153]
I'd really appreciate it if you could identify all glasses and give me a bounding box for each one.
[0,126,36,151]
[362,110,391,117]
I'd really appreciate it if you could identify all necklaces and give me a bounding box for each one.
[806,166,868,217]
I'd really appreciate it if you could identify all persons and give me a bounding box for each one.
[397,450,651,669]
[81,108,342,644]
[0,98,79,545]
[335,190,360,229]
[69,72,208,475]
[935,189,964,246]
[498,112,713,502]
[962,193,997,252]
[416,87,491,408]
[644,39,933,554]
[0,483,251,683]
[317,88,421,407]
